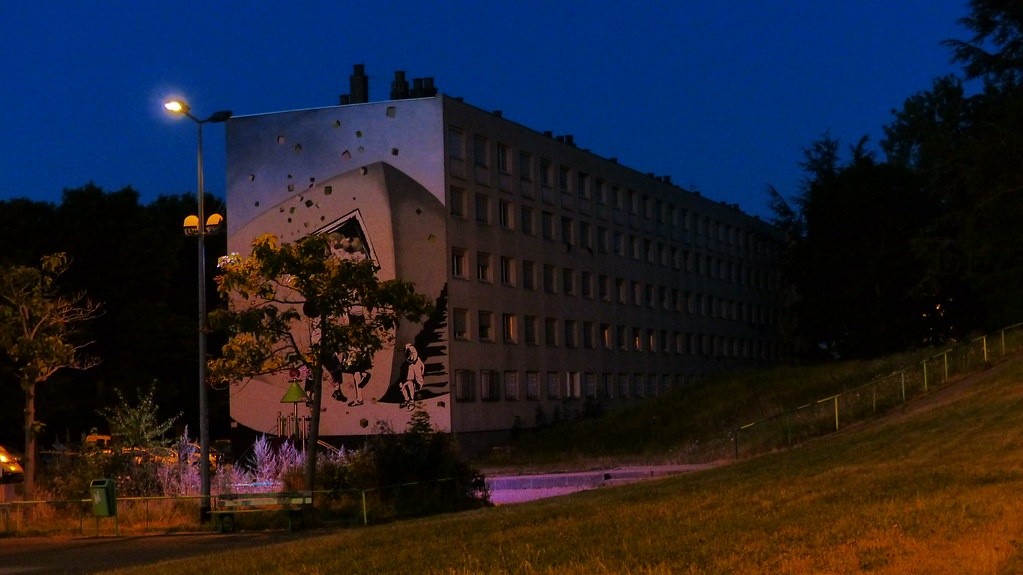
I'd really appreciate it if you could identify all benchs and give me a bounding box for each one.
[207,490,312,534]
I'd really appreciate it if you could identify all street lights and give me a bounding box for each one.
[164,100,234,525]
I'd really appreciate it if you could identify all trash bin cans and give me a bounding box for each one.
[89,478,117,516]
